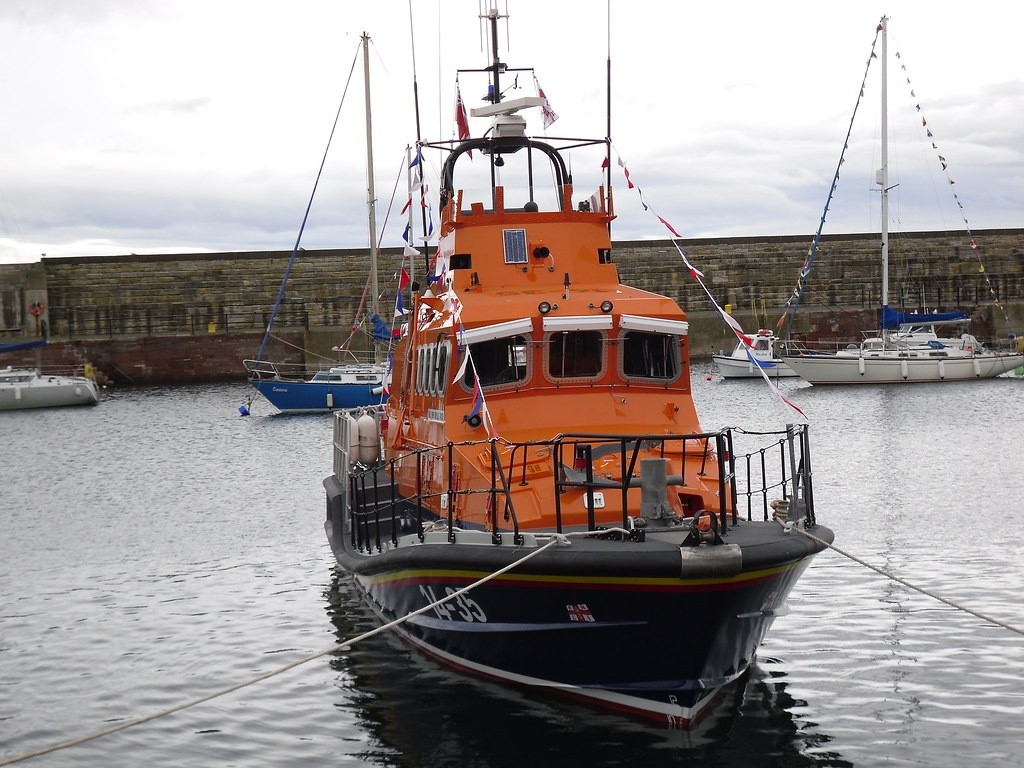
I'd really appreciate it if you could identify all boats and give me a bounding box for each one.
[712,300,800,377]
[0,341,100,410]
[243,30,394,412]
[323,0,834,728]
[774,16,1024,385]
